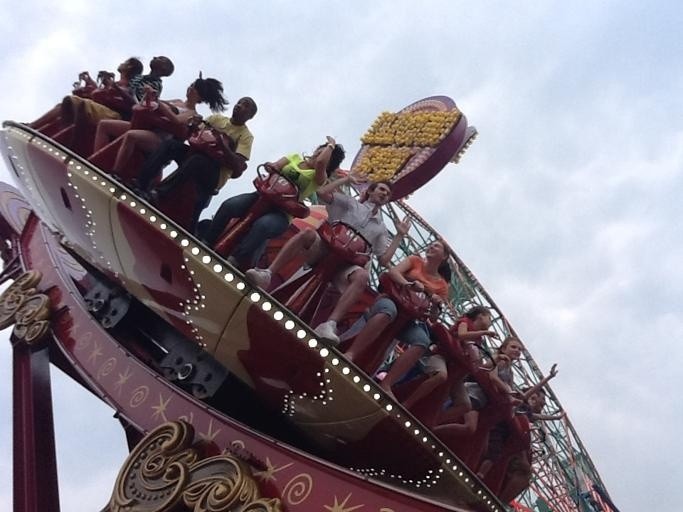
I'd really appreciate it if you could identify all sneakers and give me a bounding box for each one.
[245,267,273,291]
[314,320,340,346]
[105,169,160,209]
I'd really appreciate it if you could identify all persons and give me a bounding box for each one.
[22,56,258,231]
[198,135,565,502]
